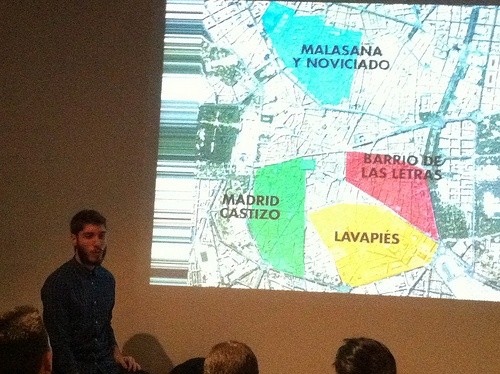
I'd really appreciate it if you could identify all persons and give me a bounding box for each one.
[204,340,259,374]
[0,304,52,374]
[41,209,150,374]
[332,337,396,374]
